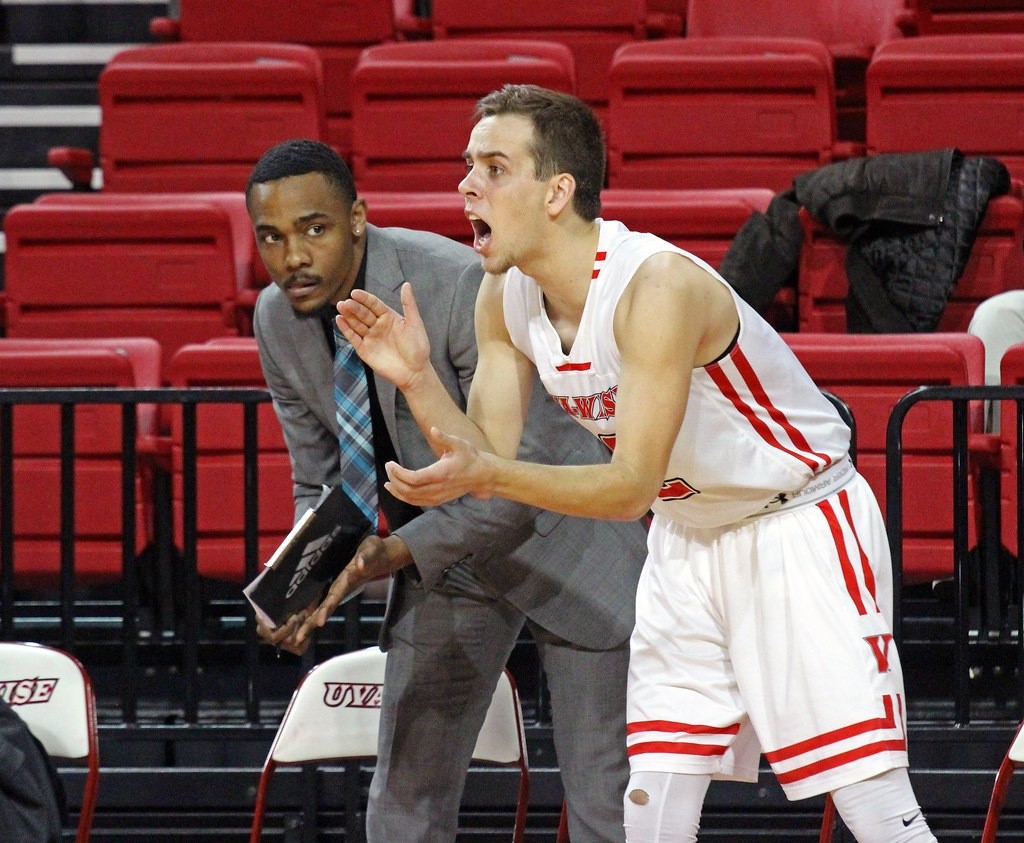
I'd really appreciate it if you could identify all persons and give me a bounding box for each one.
[331,82,942,842]
[243,139,647,838]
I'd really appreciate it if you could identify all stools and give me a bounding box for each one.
[1,0,1023,843]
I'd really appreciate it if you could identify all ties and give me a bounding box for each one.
[331,323,380,532]
